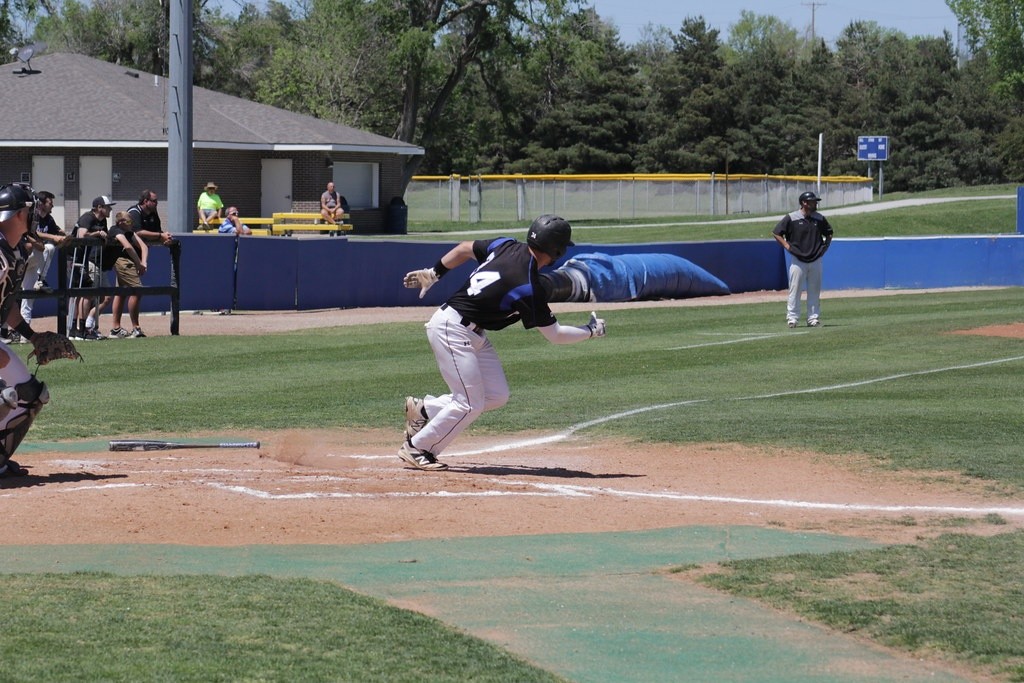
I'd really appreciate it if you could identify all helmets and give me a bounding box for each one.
[527,214,575,260]
[799,191,821,204]
[0,185,30,223]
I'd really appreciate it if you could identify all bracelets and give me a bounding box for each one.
[158,233,162,239]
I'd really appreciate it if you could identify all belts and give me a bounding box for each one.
[441,304,482,335]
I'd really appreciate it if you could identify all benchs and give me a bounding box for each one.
[274,212,353,238]
[194,217,273,237]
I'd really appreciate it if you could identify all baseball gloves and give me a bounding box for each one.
[32,331,79,365]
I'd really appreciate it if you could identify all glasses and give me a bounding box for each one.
[148,199,158,203]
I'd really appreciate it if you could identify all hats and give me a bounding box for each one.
[93,196,116,208]
[204,183,218,191]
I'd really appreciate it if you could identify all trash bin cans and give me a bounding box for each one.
[338,196,349,234]
[385,196,407,234]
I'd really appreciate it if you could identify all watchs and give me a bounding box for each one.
[825,243,830,247]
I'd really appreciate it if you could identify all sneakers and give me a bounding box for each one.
[403,395,428,441]
[398,439,448,470]
[108,328,131,339]
[69,329,75,340]
[75,329,96,341]
[87,328,106,339]
[132,328,147,338]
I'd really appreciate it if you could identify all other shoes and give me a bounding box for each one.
[808,320,821,327]
[0,337,12,344]
[0,466,28,477]
[9,331,31,343]
[789,323,797,329]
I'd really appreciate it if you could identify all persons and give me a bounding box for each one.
[84,211,148,339]
[218,207,253,235]
[197,182,224,230]
[321,183,344,225]
[397,214,606,471]
[110,188,174,340]
[0,185,78,476]
[772,192,834,328]
[64,195,116,342]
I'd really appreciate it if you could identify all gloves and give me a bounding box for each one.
[587,311,606,339]
[403,266,440,299]
